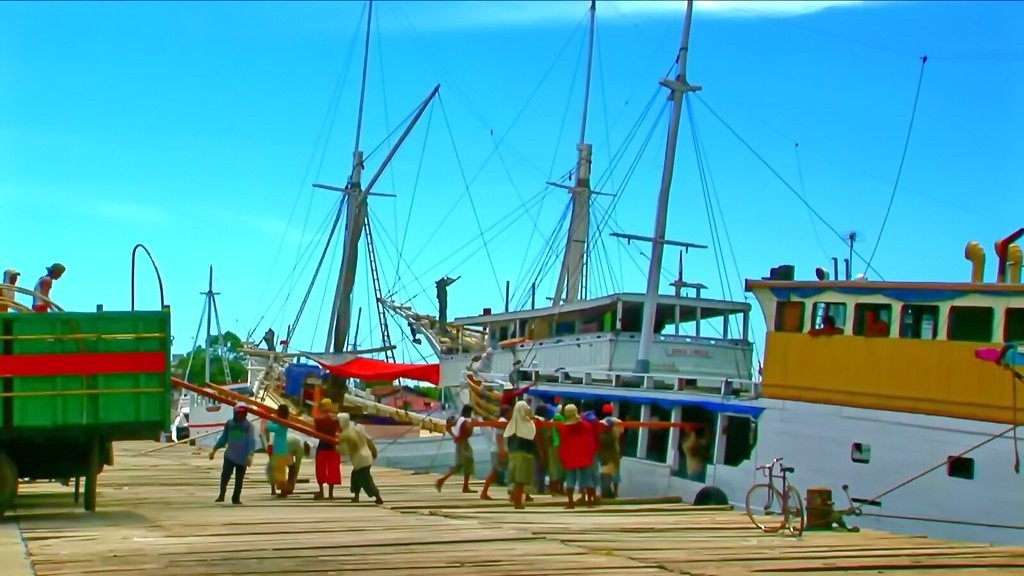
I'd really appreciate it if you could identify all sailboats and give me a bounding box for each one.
[375,0,771,498]
[174,1,505,482]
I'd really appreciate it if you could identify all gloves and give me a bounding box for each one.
[248,454,253,467]
[209,447,216,459]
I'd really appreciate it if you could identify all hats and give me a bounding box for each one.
[321,398,332,409]
[46,263,65,273]
[602,404,613,414]
[236,406,247,413]
[12,272,20,275]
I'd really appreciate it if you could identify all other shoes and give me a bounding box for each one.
[214,495,225,502]
[435,481,443,492]
[232,496,242,504]
[464,486,479,493]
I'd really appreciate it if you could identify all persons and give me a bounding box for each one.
[0,263,66,314]
[209,402,255,503]
[266,398,384,504]
[436,401,708,508]
[865,307,890,338]
[807,315,845,337]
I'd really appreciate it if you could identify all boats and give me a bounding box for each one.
[703,227,1024,545]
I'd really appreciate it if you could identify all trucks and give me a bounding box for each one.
[0,305,174,520]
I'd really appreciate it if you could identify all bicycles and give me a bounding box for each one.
[745,457,805,537]
